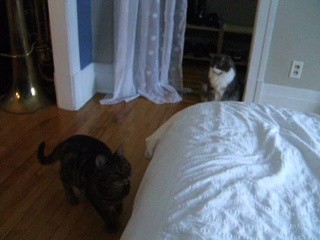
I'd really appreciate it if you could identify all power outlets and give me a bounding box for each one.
[289,61,304,79]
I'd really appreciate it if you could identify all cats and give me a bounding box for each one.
[37,134,132,232]
[177,53,244,102]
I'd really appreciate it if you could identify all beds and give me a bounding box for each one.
[121,100,320,240]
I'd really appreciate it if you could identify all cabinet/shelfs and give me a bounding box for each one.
[183,22,253,89]
